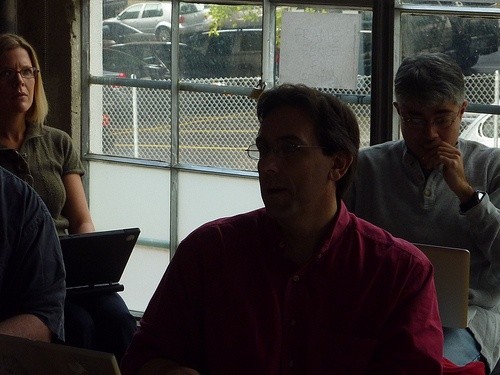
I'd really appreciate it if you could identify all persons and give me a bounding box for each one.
[121,83,443,375]
[0,165,67,346]
[0,33,138,375]
[342,52,499,374]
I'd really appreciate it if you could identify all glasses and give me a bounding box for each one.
[246,138,323,160]
[0,65,36,81]
[398,103,462,129]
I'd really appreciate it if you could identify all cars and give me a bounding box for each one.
[102,0,500,114]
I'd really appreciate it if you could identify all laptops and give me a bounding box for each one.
[412,243,477,329]
[58,228,140,296]
[0,333,121,375]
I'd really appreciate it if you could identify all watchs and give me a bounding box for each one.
[459,189,486,212]
